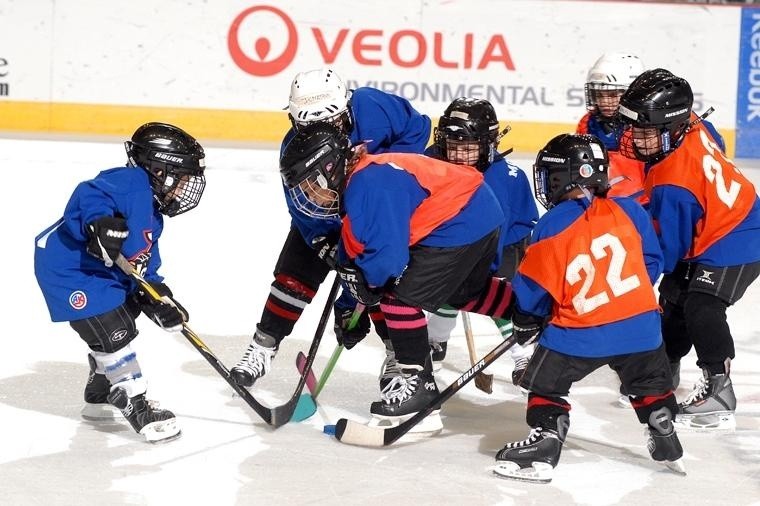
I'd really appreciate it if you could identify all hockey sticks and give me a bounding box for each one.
[127,267,317,427]
[461,311,493,393]
[115,252,366,428]
[294,302,366,401]
[272,270,342,427]
[335,335,517,449]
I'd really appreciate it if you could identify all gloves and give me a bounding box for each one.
[85,217,130,265]
[138,284,190,333]
[509,304,542,347]
[334,289,371,350]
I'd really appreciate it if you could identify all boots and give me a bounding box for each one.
[676,357,738,416]
[227,322,280,387]
[428,339,450,365]
[107,386,177,433]
[377,337,402,397]
[512,354,532,387]
[495,410,570,469]
[645,406,684,464]
[81,354,113,405]
[370,353,443,418]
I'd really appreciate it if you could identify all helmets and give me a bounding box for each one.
[431,97,514,171]
[582,54,645,122]
[531,132,611,211]
[604,69,696,161]
[124,120,207,217]
[282,68,355,136]
[279,120,356,220]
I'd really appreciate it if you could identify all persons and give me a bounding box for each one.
[33,122,206,444]
[232,50,758,481]
